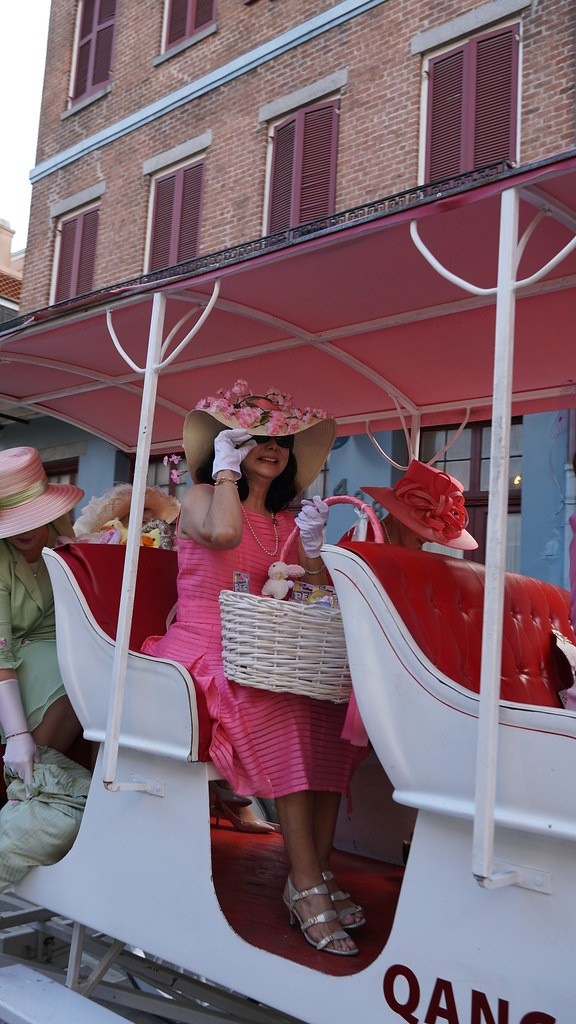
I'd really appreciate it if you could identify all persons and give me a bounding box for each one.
[75,484,274,833]
[0,445,99,783]
[329,459,478,746]
[141,379,366,954]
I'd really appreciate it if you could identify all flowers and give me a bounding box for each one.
[162,377,334,484]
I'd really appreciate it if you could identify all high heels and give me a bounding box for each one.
[211,782,253,806]
[282,874,359,955]
[216,805,275,833]
[322,872,366,929]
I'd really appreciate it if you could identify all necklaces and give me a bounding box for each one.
[241,502,278,556]
[381,521,391,544]
[31,557,42,576]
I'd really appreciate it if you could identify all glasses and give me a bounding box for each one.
[253,435,294,449]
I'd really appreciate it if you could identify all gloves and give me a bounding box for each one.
[294,495,329,558]
[0,679,41,797]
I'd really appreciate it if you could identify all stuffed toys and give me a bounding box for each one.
[262,562,305,599]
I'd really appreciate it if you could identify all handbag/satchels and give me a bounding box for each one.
[212,429,256,481]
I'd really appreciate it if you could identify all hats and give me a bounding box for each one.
[0,446,84,541]
[73,481,180,536]
[360,460,479,550]
[182,378,337,494]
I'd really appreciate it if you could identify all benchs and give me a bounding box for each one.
[319,543,576,840]
[39,541,228,780]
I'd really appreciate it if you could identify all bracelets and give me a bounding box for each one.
[305,564,325,574]
[6,731,30,740]
[213,477,238,488]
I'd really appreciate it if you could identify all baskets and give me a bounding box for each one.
[219,495,384,703]
